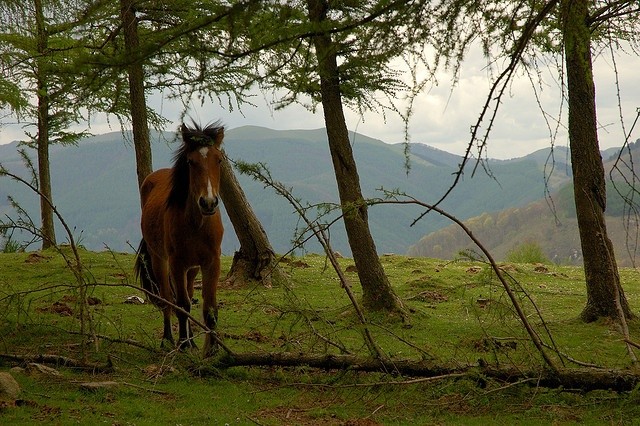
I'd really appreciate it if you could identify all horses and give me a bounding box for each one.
[132,116,228,358]
